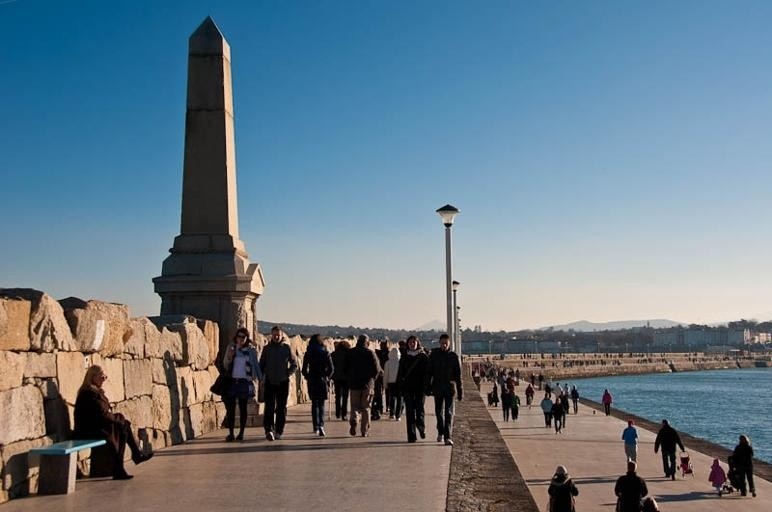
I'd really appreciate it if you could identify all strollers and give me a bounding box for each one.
[677,450,694,478]
[484,390,495,408]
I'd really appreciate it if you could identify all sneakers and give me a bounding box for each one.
[265,431,282,441]
[446,438,454,446]
[437,433,444,442]
[313,426,325,436]
[349,425,372,437]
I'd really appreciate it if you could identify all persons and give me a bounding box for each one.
[73,364,154,480]
[733,435,757,497]
[654,419,685,481]
[614,461,648,512]
[548,464,580,512]
[621,419,638,463]
[708,458,727,497]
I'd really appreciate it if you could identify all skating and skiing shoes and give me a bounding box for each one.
[226,434,245,442]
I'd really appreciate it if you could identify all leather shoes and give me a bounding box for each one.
[112,473,136,480]
[132,451,154,465]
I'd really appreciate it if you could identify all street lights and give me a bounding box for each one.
[435,203,463,366]
[488,340,728,356]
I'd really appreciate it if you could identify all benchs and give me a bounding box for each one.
[29,438,107,494]
